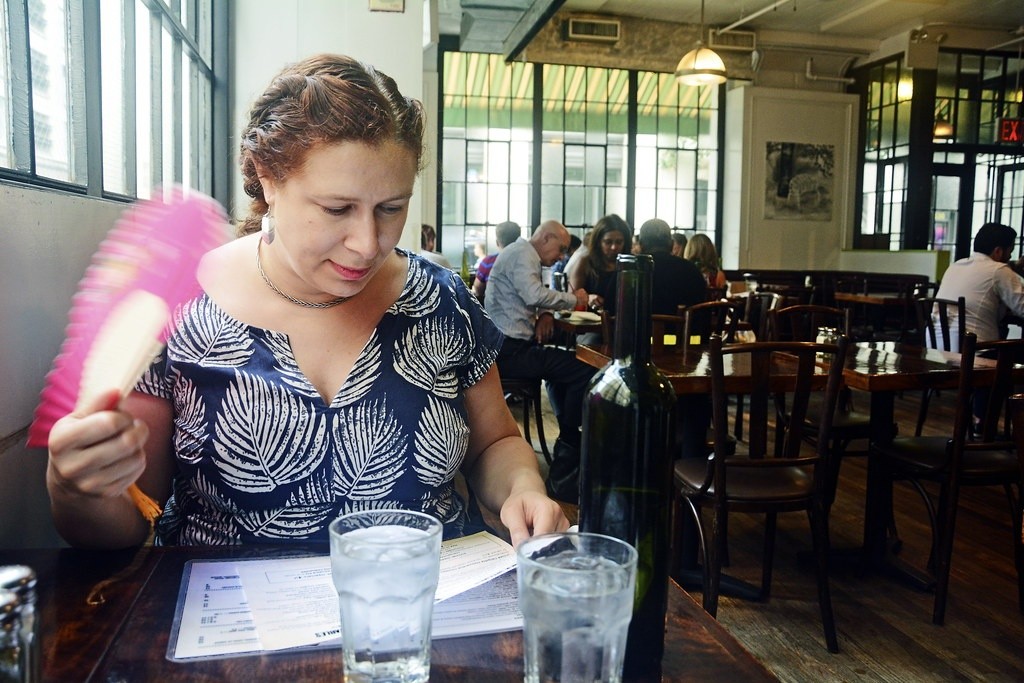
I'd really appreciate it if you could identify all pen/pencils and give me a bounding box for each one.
[567,281,575,292]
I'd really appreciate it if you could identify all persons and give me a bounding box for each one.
[44,54,574,552]
[421,214,727,315]
[924,221,1024,439]
[483,219,590,506]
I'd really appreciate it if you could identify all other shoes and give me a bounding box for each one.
[973,423,982,439]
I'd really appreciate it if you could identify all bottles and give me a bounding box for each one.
[815,327,838,361]
[576,251,679,683]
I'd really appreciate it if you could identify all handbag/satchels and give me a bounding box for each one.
[544,405,579,505]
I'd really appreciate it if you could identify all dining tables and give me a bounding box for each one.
[583,331,833,584]
[770,332,1023,579]
[837,288,924,341]
[3,537,783,683]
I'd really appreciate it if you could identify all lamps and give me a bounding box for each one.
[674,1,731,89]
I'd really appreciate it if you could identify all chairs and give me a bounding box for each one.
[905,292,1001,444]
[673,336,854,656]
[681,300,789,506]
[852,330,1024,624]
[771,303,896,504]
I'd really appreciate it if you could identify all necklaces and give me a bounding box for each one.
[255,235,355,309]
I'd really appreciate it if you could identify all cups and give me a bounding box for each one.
[328,509,443,683]
[515,531,639,683]
